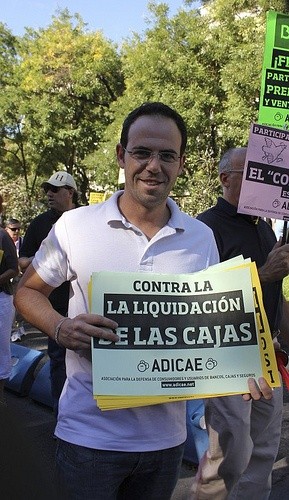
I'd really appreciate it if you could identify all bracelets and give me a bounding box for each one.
[54,317,69,349]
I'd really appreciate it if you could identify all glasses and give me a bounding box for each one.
[8,227,20,231]
[122,145,182,164]
[43,184,68,193]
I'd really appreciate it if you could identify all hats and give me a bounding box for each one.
[40,171,77,192]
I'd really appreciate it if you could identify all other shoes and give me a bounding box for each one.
[18,326,27,336]
[10,327,22,342]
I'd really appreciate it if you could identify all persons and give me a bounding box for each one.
[13,102,274,500]
[18,171,84,440]
[0,195,25,391]
[190,146,289,500]
